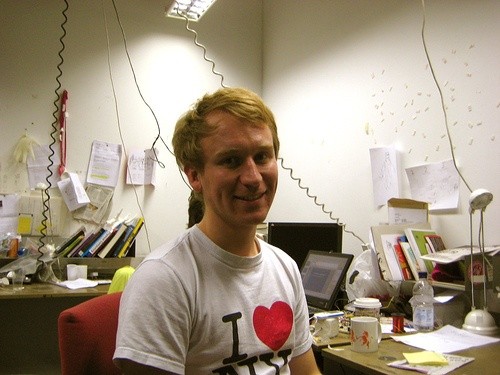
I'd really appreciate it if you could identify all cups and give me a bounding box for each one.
[350,316,382,352]
[12,267,25,286]
[353,297,382,324]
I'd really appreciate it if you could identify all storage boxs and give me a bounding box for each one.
[51,256,131,282]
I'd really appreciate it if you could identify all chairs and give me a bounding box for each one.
[55,291,124,375]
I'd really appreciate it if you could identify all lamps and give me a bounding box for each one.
[161,0,218,23]
[462,187,498,335]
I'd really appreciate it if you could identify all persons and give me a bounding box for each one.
[115,89,325,375]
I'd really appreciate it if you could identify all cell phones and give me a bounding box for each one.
[314,310,344,318]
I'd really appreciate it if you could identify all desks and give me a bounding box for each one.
[308,311,411,351]
[0,276,111,300]
[321,333,500,375]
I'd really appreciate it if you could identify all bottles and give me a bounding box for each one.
[412,272,435,332]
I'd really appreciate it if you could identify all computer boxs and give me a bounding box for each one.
[256,221,342,269]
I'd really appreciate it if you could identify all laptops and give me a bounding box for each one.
[299,249,354,315]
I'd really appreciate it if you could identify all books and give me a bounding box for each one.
[381,228,446,282]
[50,218,145,258]
